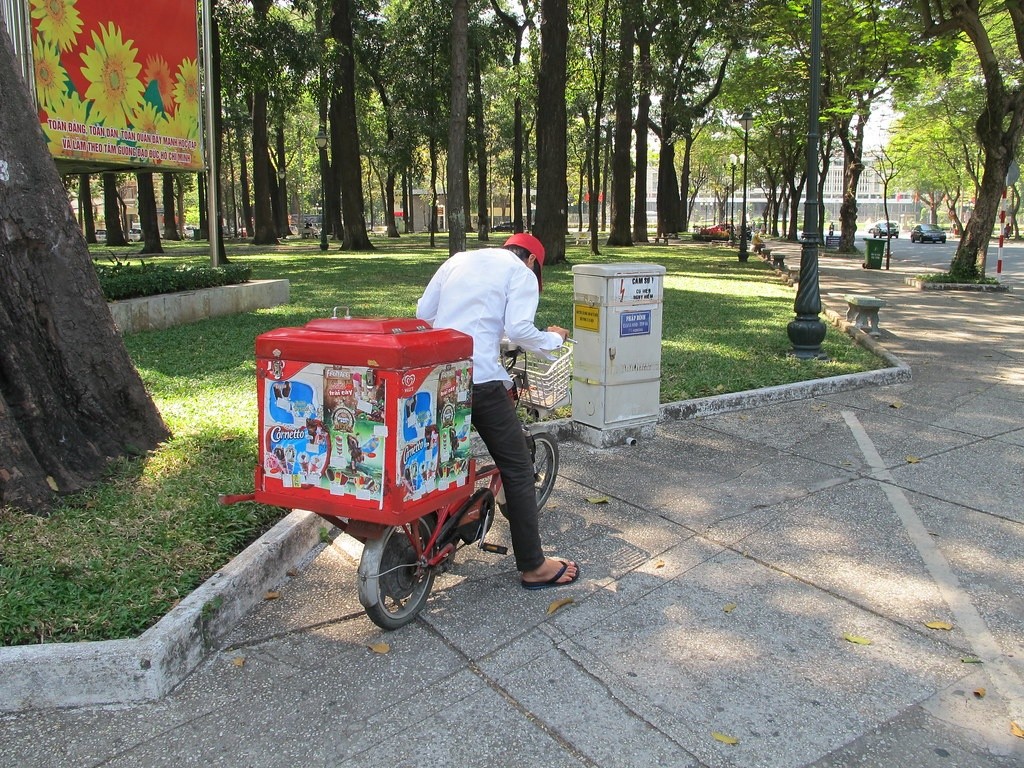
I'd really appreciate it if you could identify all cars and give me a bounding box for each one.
[490,219,514,232]
[184,225,198,238]
[911,223,948,244]
[223,225,248,239]
[122,228,141,242]
[95,229,107,242]
[868,221,900,239]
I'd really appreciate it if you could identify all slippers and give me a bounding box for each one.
[522,561,579,590]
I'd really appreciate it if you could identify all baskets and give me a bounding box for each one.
[504,345,572,410]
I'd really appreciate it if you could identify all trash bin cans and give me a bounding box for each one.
[862,237,888,269]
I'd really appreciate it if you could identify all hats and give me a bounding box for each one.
[503,233,545,291]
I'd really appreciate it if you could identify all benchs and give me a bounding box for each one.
[844,293,885,340]
[298,228,319,239]
[754,244,786,269]
[573,232,592,246]
[653,237,671,246]
[419,231,431,236]
[825,236,841,249]
[711,240,729,249]
[370,226,388,238]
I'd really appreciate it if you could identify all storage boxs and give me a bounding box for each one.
[252,318,477,528]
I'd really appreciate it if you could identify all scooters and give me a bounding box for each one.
[220,306,579,633]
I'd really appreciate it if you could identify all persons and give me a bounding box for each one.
[1005,223,1011,238]
[416,233,579,590]
[829,222,834,235]
[751,220,766,254]
[305,223,319,238]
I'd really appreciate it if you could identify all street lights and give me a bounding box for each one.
[278,167,288,238]
[721,153,746,249]
[314,124,331,250]
[737,106,755,263]
[721,176,732,230]
[316,203,321,228]
